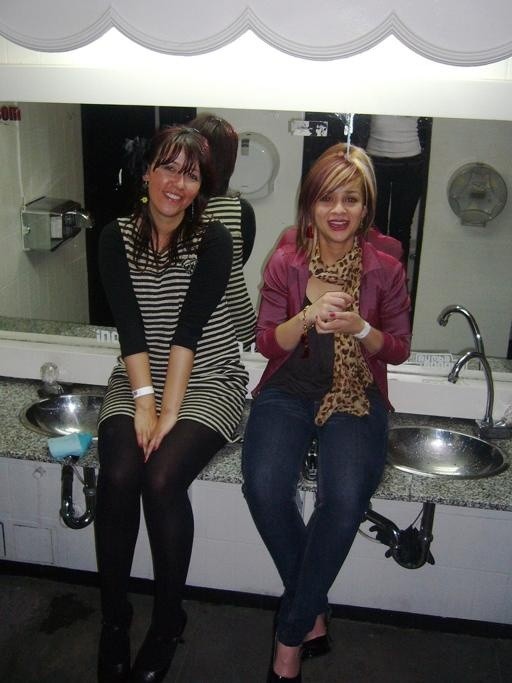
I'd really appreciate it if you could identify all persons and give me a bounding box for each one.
[186,114,259,354]
[242,153,412,683]
[276,143,407,276]
[364,115,425,271]
[95,124,249,683]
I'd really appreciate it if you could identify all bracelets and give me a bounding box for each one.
[302,305,315,359]
[132,386,155,399]
[353,321,371,339]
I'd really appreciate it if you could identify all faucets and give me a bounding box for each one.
[435,304,486,373]
[448,349,496,438]
[35,362,67,399]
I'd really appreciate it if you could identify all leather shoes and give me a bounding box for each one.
[96,608,135,683]
[132,603,188,683]
[267,631,303,683]
[302,607,333,659]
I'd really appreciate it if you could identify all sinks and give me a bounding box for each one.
[383,421,509,480]
[20,392,105,443]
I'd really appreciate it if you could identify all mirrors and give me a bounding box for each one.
[1,100,512,378]
